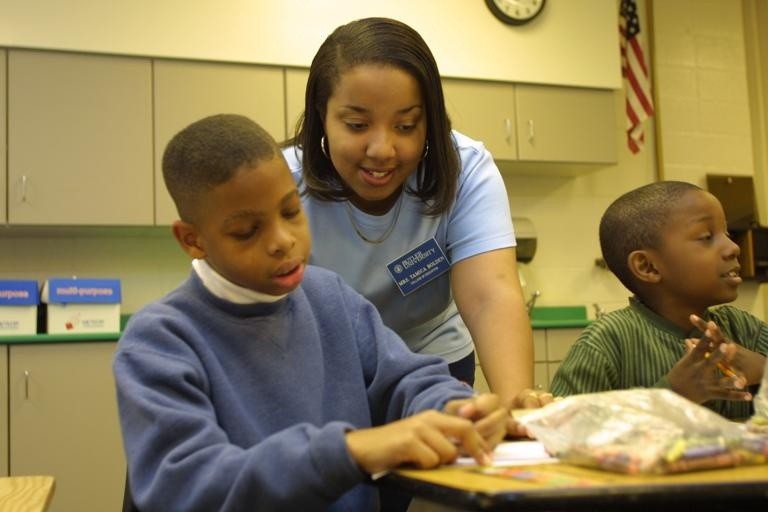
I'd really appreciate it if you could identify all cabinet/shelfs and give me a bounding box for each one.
[286,65,620,178]
[0,45,286,226]
[0,333,132,512]
[474,326,585,395]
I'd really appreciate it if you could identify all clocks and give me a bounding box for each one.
[483,0,547,26]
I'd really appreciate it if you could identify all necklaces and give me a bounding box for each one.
[338,181,406,244]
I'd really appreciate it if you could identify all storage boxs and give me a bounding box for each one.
[38,277,123,334]
[0,279,39,337]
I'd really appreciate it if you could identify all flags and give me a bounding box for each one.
[618,2,655,157]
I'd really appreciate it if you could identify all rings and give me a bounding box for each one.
[520,393,539,410]
[540,393,552,404]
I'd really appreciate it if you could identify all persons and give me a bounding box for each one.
[113,113,507,511]
[269,17,552,441]
[547,182,768,421]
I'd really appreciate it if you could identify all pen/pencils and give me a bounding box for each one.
[684,339,738,380]
[371,435,461,481]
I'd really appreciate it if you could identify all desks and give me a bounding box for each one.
[0,474,56,512]
[391,402,768,512]
